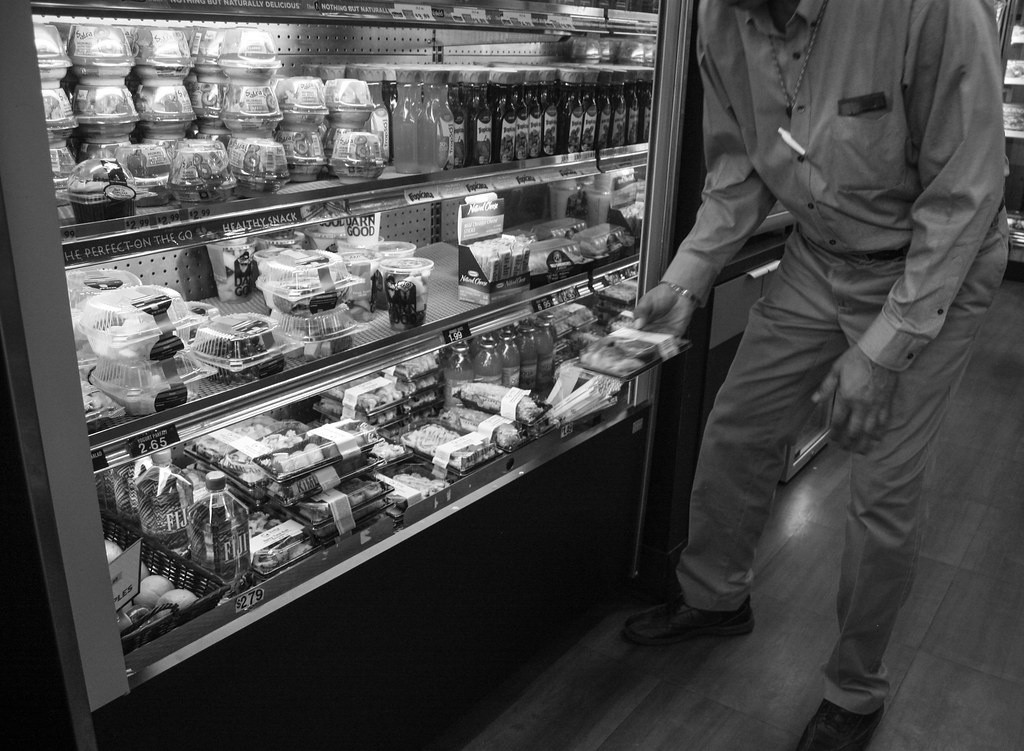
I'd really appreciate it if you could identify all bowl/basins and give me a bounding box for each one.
[572,36,657,67]
[33,24,384,223]
[65,247,691,582]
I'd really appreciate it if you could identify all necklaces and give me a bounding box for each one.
[768,0,827,116]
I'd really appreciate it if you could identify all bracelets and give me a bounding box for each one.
[670,283,697,301]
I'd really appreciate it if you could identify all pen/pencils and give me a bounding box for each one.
[778,126,805,156]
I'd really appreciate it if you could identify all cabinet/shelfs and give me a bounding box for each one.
[996,0,1024,282]
[1,0,846,750]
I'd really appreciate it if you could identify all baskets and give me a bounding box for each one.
[101,509,233,654]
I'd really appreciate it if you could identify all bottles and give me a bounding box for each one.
[444,344,473,407]
[136,448,196,563]
[497,332,521,388]
[474,339,502,387]
[186,472,255,607]
[112,457,153,522]
[535,311,554,338]
[383,78,651,174]
[95,469,115,508]
[534,321,553,387]
[513,326,537,392]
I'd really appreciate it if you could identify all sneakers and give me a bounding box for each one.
[625,594,754,646]
[796,694,885,751]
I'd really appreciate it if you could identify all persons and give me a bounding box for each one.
[634,0,1010,751]
